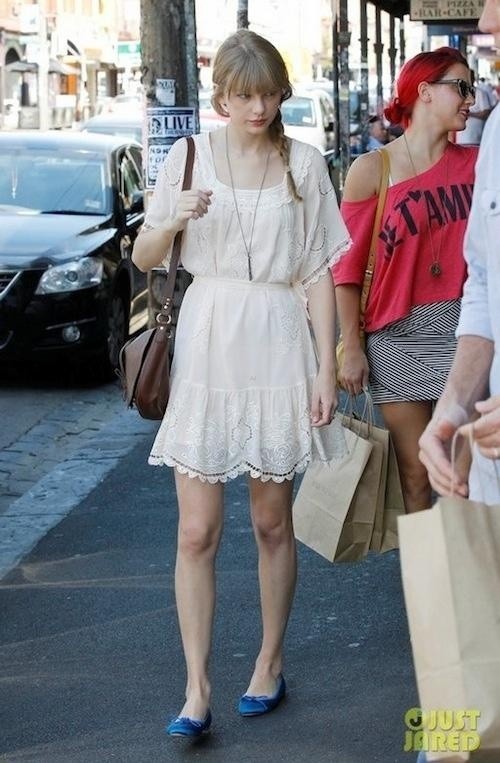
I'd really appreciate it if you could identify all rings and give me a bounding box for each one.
[331,414,335,419]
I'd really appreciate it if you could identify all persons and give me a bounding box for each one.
[130,25,355,740]
[477,77,499,109]
[364,116,390,149]
[453,69,491,147]
[330,45,481,514]
[413,0,498,763]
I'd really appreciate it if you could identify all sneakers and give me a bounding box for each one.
[164,702,213,742]
[238,673,288,719]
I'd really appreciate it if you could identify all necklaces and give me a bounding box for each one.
[223,122,273,279]
[403,133,450,277]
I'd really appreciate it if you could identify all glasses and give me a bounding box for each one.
[431,77,476,100]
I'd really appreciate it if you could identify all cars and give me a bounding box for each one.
[0,86,360,384]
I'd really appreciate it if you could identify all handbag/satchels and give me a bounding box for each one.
[394,417,499,763]
[333,323,371,392]
[115,310,173,421]
[289,387,387,567]
[327,386,409,553]
